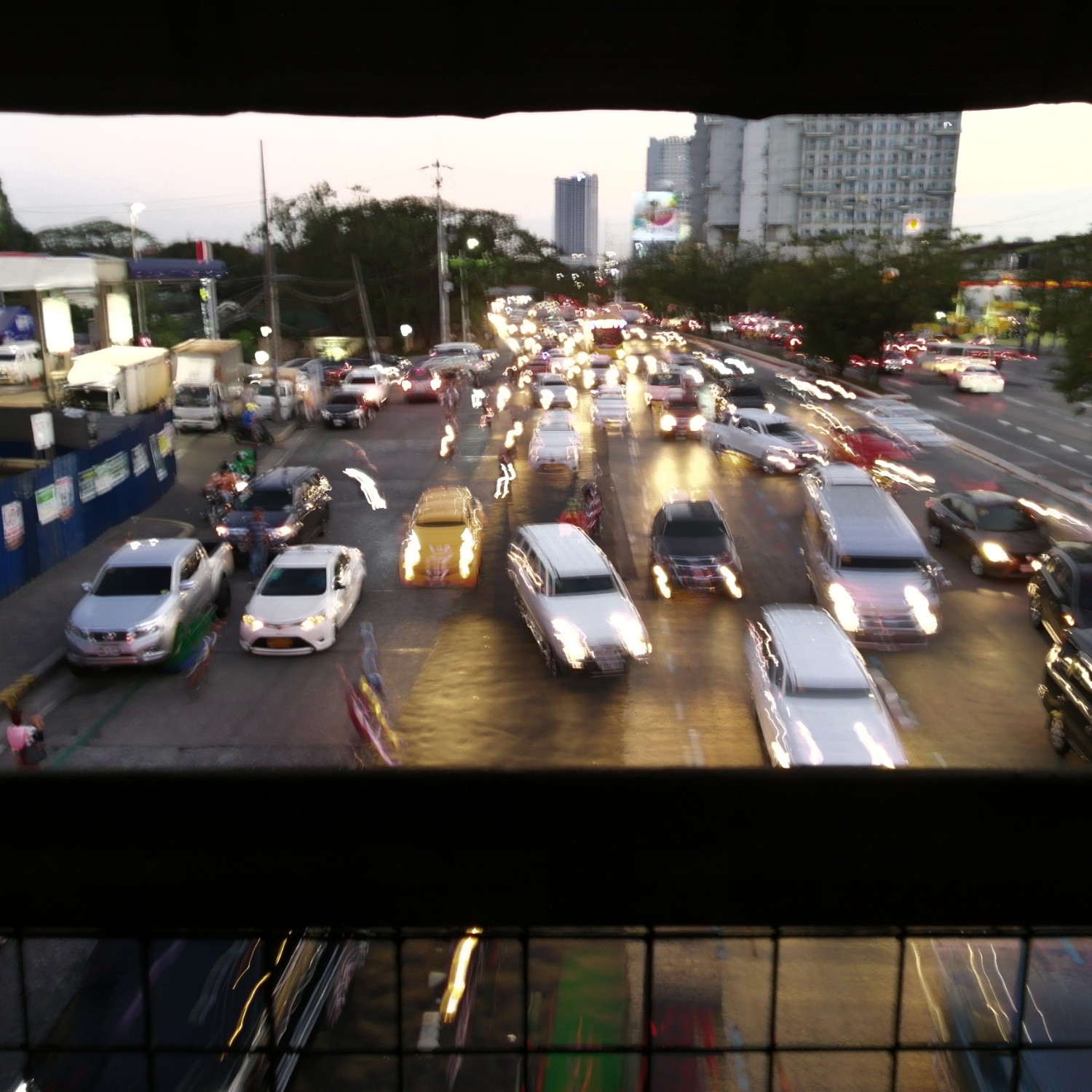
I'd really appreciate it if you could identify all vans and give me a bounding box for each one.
[341,358,399,378]
[339,366,392,410]
[0,340,43,386]
[506,522,651,674]
[800,478,945,649]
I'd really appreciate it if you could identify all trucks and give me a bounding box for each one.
[60,345,171,439]
[255,359,321,420]
[170,339,244,434]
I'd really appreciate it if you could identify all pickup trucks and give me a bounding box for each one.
[62,536,237,673]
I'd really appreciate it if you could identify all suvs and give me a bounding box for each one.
[646,499,744,597]
[428,344,500,364]
[216,465,331,563]
[1039,627,1092,761]
[402,368,446,402]
[1026,541,1091,658]
[320,391,373,429]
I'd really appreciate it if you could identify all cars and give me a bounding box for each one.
[459,355,493,375]
[322,366,351,385]
[399,486,485,587]
[423,355,470,378]
[924,489,1050,577]
[364,353,412,372]
[238,543,367,657]
[488,294,1013,471]
[742,604,908,767]
